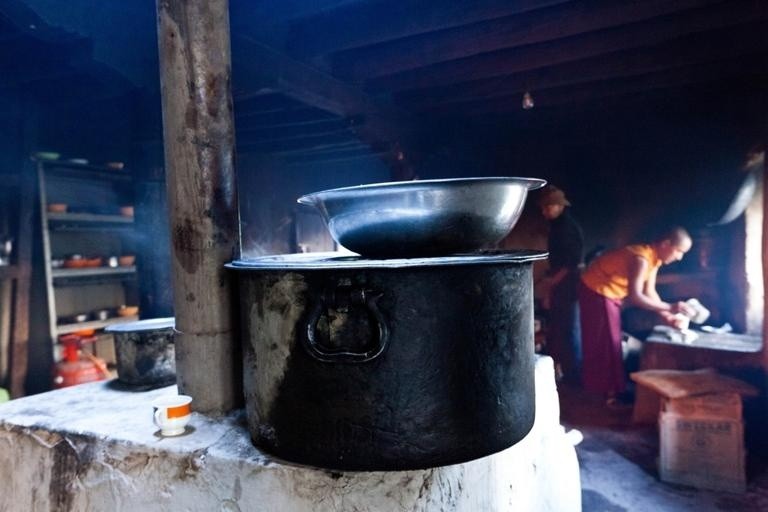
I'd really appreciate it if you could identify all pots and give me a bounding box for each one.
[98,316,176,389]
[218,248,558,475]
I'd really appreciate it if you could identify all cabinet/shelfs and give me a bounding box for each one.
[27,155,138,389]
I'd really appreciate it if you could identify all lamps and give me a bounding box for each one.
[522,92,534,109]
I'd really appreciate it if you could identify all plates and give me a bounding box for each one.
[33,150,125,169]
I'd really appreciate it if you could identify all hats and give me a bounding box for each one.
[535,184,571,207]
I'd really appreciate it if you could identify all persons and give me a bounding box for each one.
[577,223,697,411]
[532,183,584,380]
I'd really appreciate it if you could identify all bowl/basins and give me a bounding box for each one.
[57,301,142,323]
[47,200,69,215]
[120,204,136,218]
[47,248,139,271]
[291,170,553,260]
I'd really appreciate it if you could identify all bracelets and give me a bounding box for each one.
[664,313,673,322]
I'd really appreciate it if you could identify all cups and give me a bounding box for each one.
[681,298,710,325]
[674,312,690,329]
[149,393,196,438]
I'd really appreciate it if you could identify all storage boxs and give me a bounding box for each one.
[659,395,746,497]
